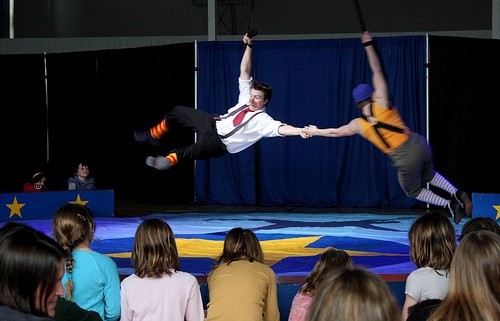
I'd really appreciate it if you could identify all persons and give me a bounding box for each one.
[401,213,457,321]
[126,32,312,170]
[309,268,403,321]
[69,160,98,190]
[288,249,353,321]
[24,170,49,192]
[120,218,205,321]
[52,203,120,321]
[459,217,500,241]
[304,32,473,225]
[205,227,281,321]
[406,230,500,321]
[0,221,103,321]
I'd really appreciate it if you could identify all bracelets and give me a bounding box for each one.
[362,41,373,47]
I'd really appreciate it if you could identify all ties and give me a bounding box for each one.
[233,108,254,126]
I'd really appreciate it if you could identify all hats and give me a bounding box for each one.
[352,84,375,102]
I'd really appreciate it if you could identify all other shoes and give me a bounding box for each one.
[133,129,159,146]
[456,190,472,216]
[143,155,172,178]
[449,200,463,225]
